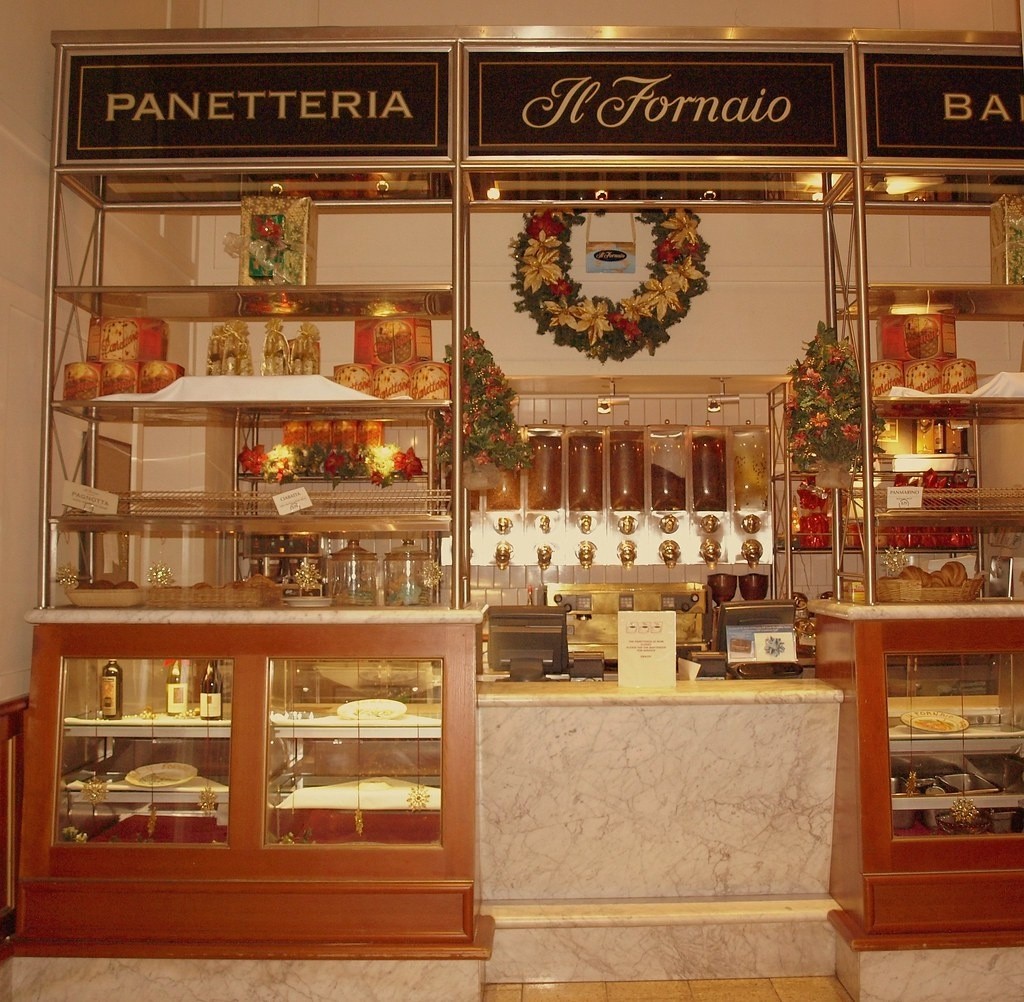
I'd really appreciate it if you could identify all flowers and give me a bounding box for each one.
[782,321,887,474]
[432,324,536,471]
[507,206,711,367]
[238,441,423,489]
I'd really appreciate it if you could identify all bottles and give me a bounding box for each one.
[933,419,947,454]
[101,659,123,720]
[199,660,223,721]
[330,540,380,607]
[383,539,431,608]
[164,660,189,717]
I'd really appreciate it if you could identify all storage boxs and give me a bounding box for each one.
[903,359,941,395]
[870,359,905,397]
[988,192,1024,286]
[903,313,957,360]
[237,194,318,287]
[941,358,979,394]
[353,316,433,369]
[85,315,170,363]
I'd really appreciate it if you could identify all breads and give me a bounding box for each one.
[881,560,969,587]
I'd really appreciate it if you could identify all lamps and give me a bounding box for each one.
[707,381,740,413]
[597,379,631,415]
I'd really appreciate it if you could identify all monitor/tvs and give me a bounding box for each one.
[717,599,796,651]
[487,605,570,683]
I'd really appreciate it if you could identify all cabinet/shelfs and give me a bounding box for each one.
[813,615,1023,953]
[765,381,984,601]
[4,619,496,962]
[35,160,468,611]
[231,406,443,605]
[822,172,1024,605]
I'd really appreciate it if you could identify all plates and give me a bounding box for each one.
[336,699,407,720]
[124,762,198,787]
[900,711,970,734]
